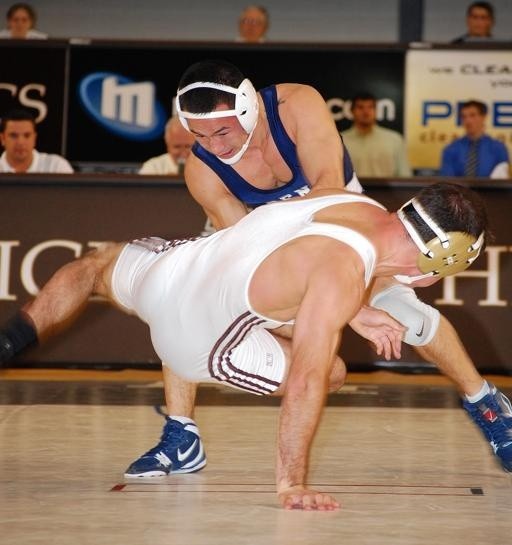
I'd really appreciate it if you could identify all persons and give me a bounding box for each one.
[451,2,495,43]
[0,182,495,511]
[138,117,195,175]
[340,90,413,179]
[0,109,74,175]
[238,6,269,42]
[122,60,512,478]
[0,3,47,39]
[441,100,508,178]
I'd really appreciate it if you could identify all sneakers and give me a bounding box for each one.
[124,421,207,478]
[462,377,512,474]
